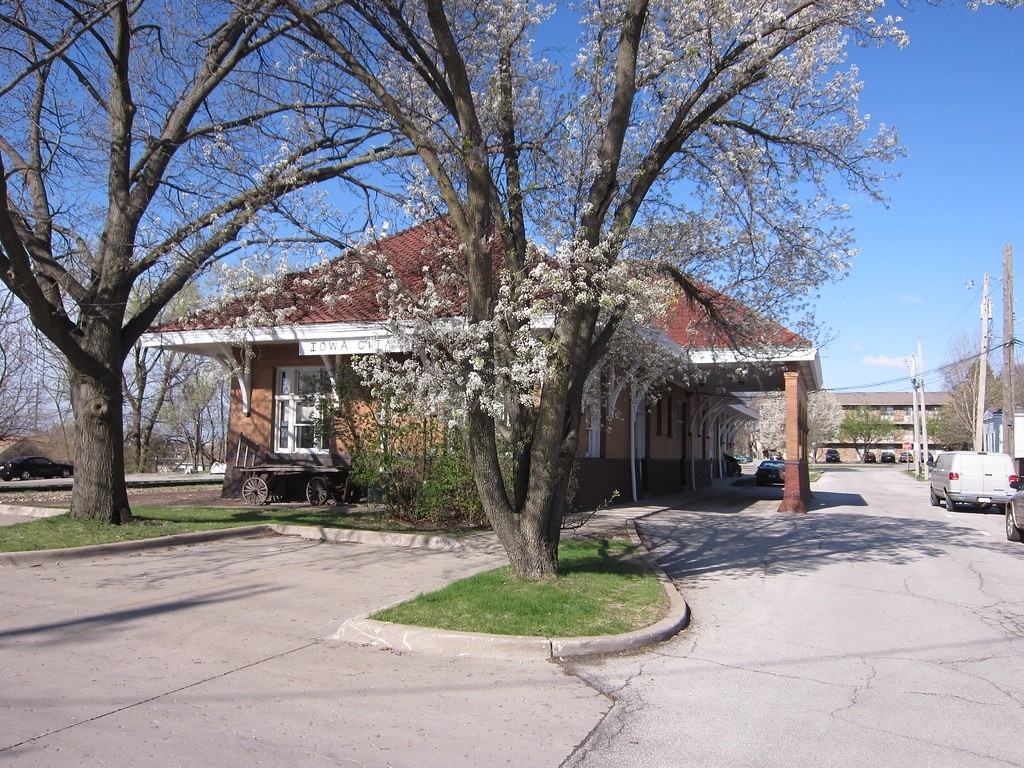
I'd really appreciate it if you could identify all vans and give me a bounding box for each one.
[926,450,1019,515]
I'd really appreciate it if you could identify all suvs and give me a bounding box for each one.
[824,449,841,463]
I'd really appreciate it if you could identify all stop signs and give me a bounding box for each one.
[903,442,912,451]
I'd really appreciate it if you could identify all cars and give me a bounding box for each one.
[755,460,785,487]
[898,452,913,463]
[0,455,73,482]
[734,454,753,464]
[769,452,784,460]
[921,450,946,464]
[880,451,896,463]
[863,452,876,463]
[1005,476,1024,543]
